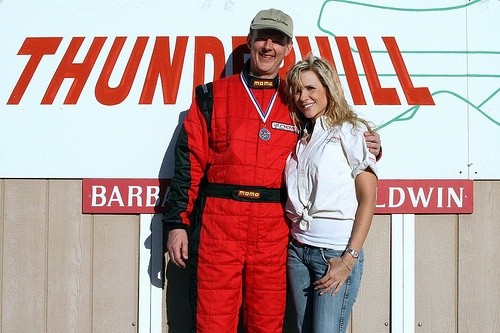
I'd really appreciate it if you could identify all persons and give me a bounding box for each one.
[162,9,382,333]
[285,50,378,333]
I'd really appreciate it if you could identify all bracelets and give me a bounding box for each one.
[341,257,352,271]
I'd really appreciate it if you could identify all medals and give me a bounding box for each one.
[259,128,271,141]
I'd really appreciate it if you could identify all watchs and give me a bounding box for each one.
[345,248,360,260]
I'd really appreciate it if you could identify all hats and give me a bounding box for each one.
[250,9,292,38]
